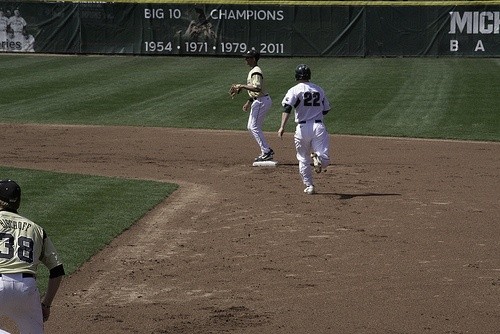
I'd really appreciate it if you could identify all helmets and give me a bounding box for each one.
[295,64,311,80]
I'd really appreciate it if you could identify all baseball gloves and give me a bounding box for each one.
[228,83,241,100]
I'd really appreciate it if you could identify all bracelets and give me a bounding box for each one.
[41,303,51,308]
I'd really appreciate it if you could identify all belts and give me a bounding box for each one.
[299,120,321,124]
[0,273,35,278]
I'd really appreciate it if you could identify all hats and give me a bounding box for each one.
[242,47,260,60]
[0,179,21,203]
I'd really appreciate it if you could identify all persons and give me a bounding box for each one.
[0,8,29,51]
[229,50,274,161]
[0,179,65,334]
[277,63,331,194]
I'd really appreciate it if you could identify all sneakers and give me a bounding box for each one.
[310,152,322,174]
[255,152,273,161]
[304,185,315,194]
